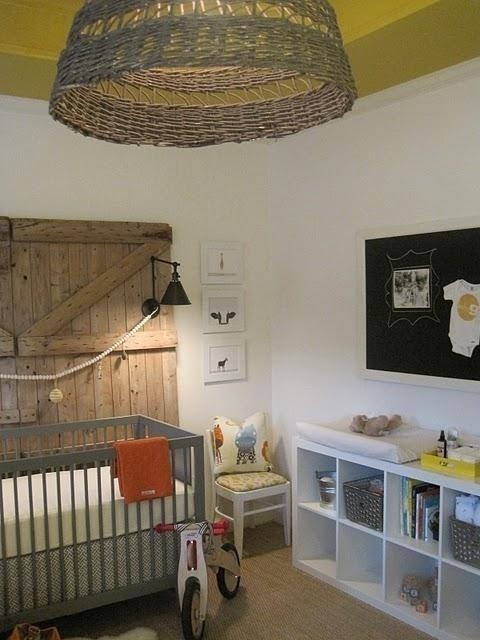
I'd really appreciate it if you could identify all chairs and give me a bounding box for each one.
[204,428,292,561]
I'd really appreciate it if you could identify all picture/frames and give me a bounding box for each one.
[197,238,250,385]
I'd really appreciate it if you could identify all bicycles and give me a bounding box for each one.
[154,505,242,640]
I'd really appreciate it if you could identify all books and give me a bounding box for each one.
[400,475,440,542]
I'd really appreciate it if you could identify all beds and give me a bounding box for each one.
[0,413,206,635]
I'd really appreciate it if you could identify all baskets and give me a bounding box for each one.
[449,514,480,569]
[343,474,383,532]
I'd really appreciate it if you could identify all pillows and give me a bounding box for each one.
[209,413,271,478]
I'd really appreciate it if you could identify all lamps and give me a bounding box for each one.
[140,255,190,317]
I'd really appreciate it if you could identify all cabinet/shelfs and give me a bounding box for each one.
[289,436,480,638]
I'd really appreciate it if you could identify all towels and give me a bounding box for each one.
[114,436,173,504]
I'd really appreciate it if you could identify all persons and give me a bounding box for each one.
[393,268,428,307]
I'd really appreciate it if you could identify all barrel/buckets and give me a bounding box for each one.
[315,470,336,510]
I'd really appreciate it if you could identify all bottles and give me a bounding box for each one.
[437,430,446,458]
